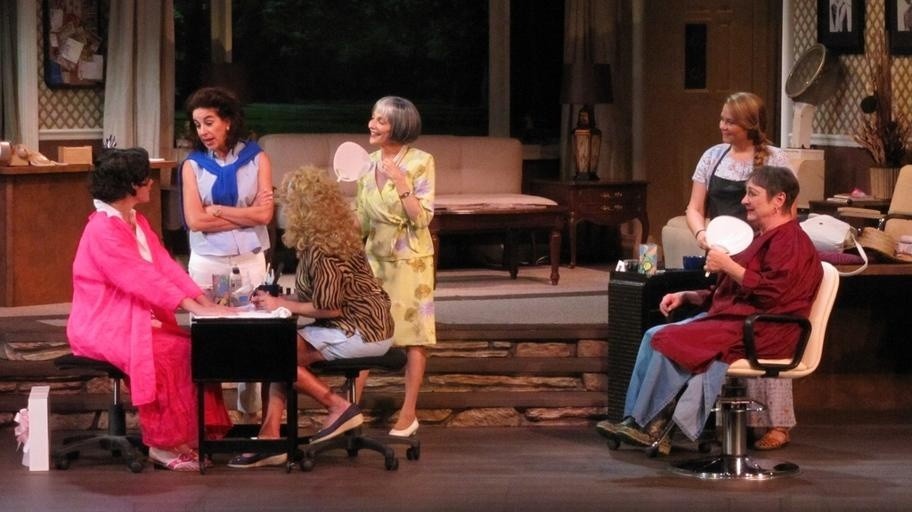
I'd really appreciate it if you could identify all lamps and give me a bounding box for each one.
[558,58,618,181]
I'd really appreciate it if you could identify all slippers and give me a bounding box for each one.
[178,446,214,469]
[146,449,200,473]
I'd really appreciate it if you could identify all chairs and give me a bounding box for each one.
[830,163,912,260]
[668,257,841,481]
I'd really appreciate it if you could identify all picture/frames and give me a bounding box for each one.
[882,0,912,58]
[814,0,864,56]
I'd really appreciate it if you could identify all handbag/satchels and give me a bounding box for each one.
[799,211,868,277]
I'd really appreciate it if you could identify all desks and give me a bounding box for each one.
[189,306,302,471]
[539,180,650,272]
[603,261,911,460]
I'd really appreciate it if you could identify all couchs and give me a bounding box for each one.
[254,126,564,290]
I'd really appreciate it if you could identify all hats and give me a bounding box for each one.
[843,225,911,265]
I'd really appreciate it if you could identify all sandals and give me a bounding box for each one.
[753,426,793,451]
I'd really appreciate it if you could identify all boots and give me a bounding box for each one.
[617,380,689,457]
[595,416,637,444]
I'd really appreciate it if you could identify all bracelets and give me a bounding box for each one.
[692,229,705,240]
[212,204,224,219]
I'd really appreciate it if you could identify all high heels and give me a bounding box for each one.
[227,451,288,469]
[308,402,364,446]
[388,416,420,439]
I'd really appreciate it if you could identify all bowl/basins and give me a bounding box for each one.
[681,255,705,270]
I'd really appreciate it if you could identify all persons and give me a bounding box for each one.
[687,91,798,452]
[66,148,231,472]
[597,166,824,457]
[226,164,395,470]
[353,96,436,438]
[176,85,275,424]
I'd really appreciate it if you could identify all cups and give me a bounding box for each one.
[212,273,229,299]
[624,259,637,272]
[639,242,658,277]
[258,284,279,297]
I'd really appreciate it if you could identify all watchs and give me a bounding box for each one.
[399,191,411,200]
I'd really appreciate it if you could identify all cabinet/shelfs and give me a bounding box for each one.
[2,158,176,312]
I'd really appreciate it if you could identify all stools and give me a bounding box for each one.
[47,353,152,475]
[299,352,422,473]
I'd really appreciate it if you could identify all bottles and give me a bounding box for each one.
[230,267,242,292]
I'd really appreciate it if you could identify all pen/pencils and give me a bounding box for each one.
[106,134,118,149]
